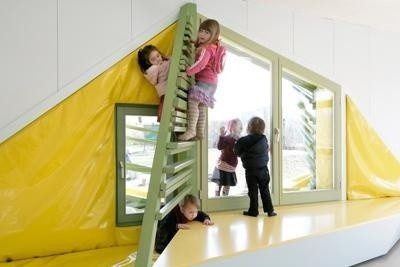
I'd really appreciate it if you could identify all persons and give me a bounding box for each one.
[137,45,184,142]
[177,19,219,141]
[233,116,277,216]
[210,118,243,196]
[155,194,214,255]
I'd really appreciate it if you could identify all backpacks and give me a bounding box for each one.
[210,42,226,73]
